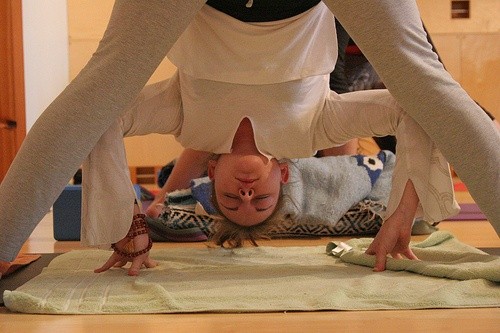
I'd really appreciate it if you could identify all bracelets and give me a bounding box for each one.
[112,237,152,257]
[132,220,147,224]
[127,229,148,239]
[130,224,148,230]
[133,213,145,219]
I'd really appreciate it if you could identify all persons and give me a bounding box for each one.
[157,12,494,198]
[0,0,500,272]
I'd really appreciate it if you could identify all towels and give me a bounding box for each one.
[3,231,500,316]
